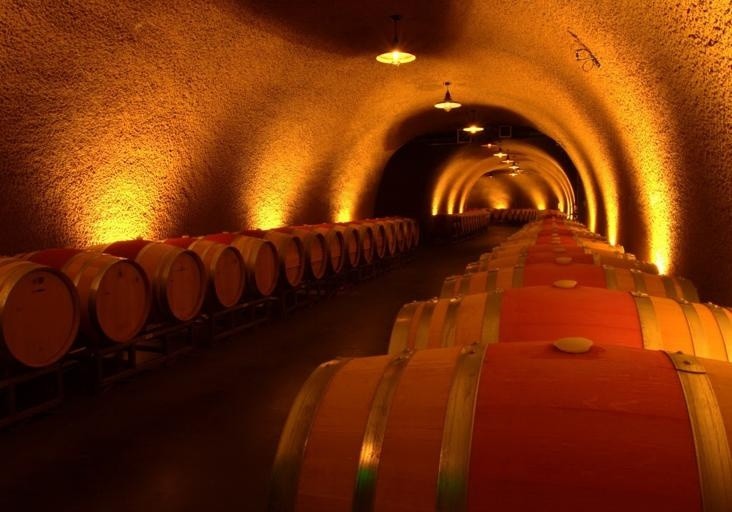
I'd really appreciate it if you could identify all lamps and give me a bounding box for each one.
[376,15,524,177]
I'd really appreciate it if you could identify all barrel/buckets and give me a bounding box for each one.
[1,204,731,512]
